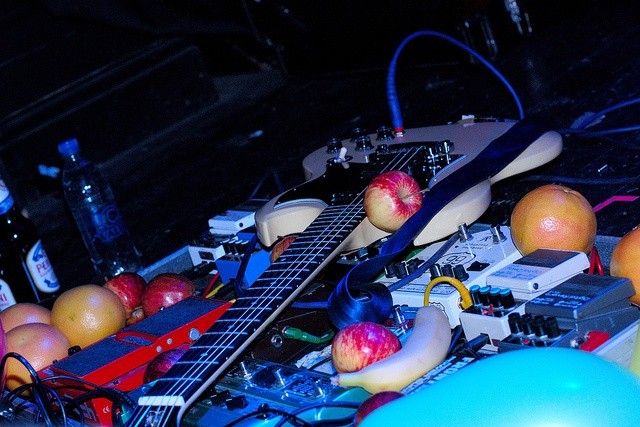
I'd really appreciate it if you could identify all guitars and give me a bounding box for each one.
[123,122,563,426]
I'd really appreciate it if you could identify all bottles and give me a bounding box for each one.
[57,137,144,283]
[0,175,65,310]
[1,254,29,312]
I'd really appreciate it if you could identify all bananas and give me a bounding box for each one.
[337,306,453,396]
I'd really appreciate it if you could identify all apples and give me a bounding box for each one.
[271,235,299,264]
[329,321,402,373]
[360,169,423,233]
[354,390,403,426]
[101,271,148,327]
[143,273,195,321]
